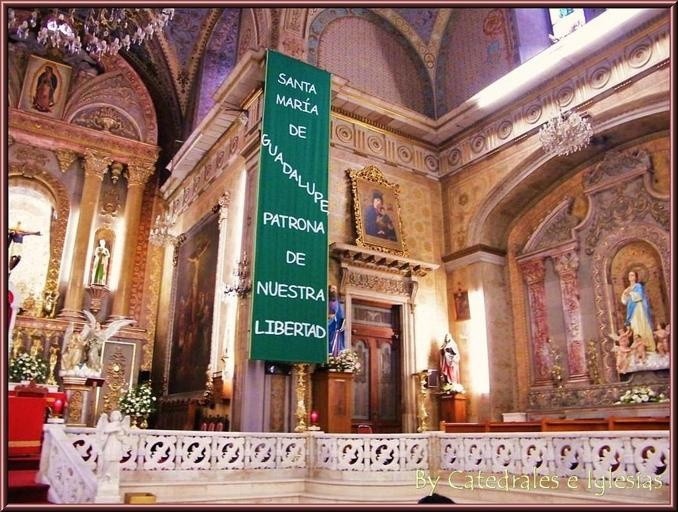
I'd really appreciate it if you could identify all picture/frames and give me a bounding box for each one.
[157,191,229,405]
[344,164,408,255]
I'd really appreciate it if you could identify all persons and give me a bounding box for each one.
[90,240,111,286]
[609,269,670,375]
[6,7,175,61]
[63,321,103,373]
[34,66,57,114]
[179,243,211,371]
[439,333,461,385]
[362,191,397,240]
[328,284,346,353]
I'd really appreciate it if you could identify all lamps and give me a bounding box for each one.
[537,99,593,157]
[6,8,175,62]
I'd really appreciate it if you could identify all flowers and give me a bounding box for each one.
[610,385,669,405]
[7,348,46,383]
[321,347,361,376]
[114,378,159,420]
